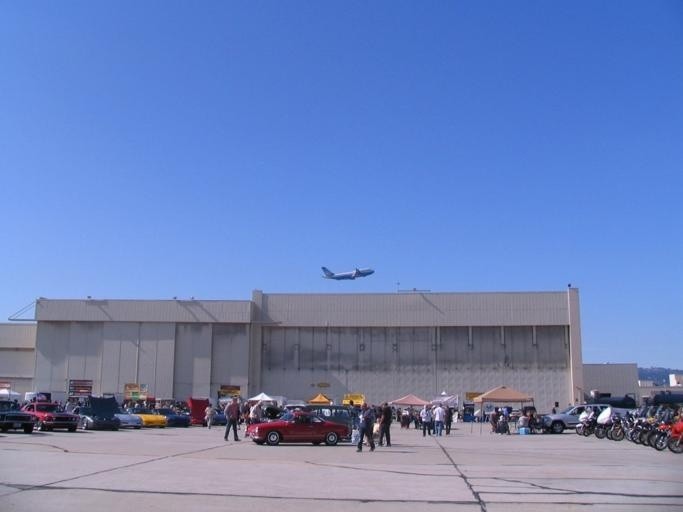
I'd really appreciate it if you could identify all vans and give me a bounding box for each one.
[303,405,359,425]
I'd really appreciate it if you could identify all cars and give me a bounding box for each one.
[0,400,191,434]
[540,402,610,434]
[202,409,227,427]
[247,411,351,446]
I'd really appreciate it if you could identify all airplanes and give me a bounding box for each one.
[321,266,375,281]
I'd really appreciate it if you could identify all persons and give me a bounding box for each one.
[491,407,541,434]
[222,399,241,441]
[122,399,192,417]
[377,403,393,446]
[349,401,453,436]
[205,404,214,429]
[13,394,82,415]
[236,399,263,431]
[356,403,375,452]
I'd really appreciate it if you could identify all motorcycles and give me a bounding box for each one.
[575,405,682,454]
